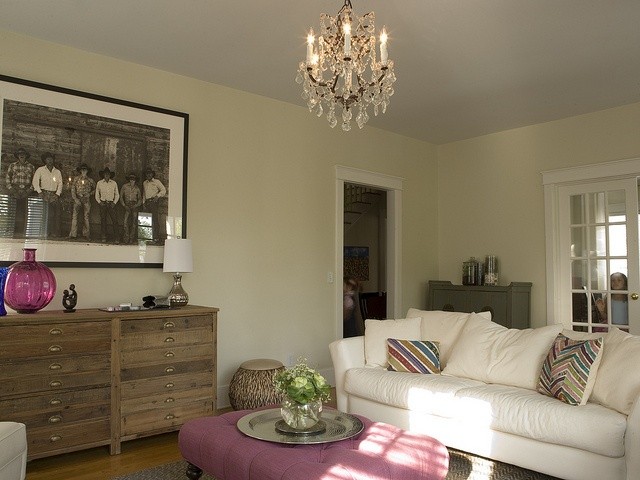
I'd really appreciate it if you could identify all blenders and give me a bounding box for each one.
[483,254,499,285]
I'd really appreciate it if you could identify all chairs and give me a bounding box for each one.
[582,285,600,321]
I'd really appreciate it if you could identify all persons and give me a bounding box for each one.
[64,163,96,241]
[95,167,119,245]
[5,147,35,202]
[33,152,63,238]
[595,272,627,330]
[120,172,141,244]
[142,167,166,245]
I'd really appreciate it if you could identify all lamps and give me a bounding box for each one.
[294,0,398,132]
[162,238,193,307]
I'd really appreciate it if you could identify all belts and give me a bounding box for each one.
[129,201,135,204]
[76,194,85,197]
[104,201,112,203]
[11,184,30,189]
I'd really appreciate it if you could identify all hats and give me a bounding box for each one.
[142,168,155,181]
[125,172,140,183]
[41,152,56,164]
[14,148,31,160]
[99,167,115,179]
[76,163,92,176]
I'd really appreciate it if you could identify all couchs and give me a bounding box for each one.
[0,421,27,480]
[327,335,640,480]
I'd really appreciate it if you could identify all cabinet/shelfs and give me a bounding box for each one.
[429,280,533,330]
[1,320,113,457]
[120,313,215,439]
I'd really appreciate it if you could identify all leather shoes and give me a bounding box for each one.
[41,189,56,194]
[148,198,153,201]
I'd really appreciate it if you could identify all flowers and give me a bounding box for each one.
[271,352,333,426]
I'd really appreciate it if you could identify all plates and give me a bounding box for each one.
[237,407,364,444]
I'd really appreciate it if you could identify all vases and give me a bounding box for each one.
[280,393,322,430]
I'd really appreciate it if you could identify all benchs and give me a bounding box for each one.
[177,403,451,480]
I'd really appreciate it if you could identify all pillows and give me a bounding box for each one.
[441,312,564,391]
[406,308,491,371]
[589,326,639,415]
[386,338,441,373]
[535,332,604,406]
[364,317,422,368]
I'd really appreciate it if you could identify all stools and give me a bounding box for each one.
[228,358,287,411]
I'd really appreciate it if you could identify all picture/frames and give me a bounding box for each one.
[0,74,190,269]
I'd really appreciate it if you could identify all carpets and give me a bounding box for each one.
[109,446,560,480]
